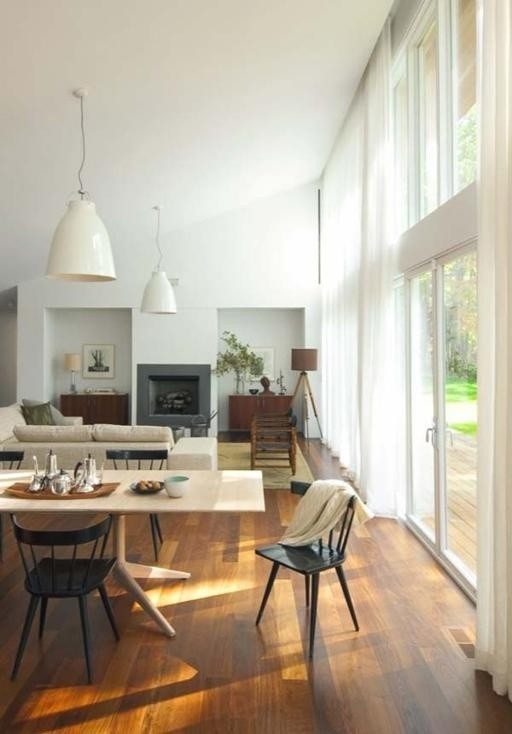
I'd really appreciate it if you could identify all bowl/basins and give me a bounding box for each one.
[249,389,258,395]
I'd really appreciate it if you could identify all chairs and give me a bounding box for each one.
[11,510,120,685]
[0,452,25,562]
[106,450,167,560]
[255,481,358,660]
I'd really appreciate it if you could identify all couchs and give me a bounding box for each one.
[1,416,218,470]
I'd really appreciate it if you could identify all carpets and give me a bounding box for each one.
[218,442,315,490]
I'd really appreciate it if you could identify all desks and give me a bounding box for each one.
[0,469,267,637]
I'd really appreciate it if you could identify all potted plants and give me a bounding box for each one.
[208,330,265,394]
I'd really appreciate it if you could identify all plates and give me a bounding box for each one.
[130,481,163,494]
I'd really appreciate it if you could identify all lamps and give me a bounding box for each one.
[65,353,82,394]
[43,86,118,283]
[289,349,324,447]
[140,205,178,314]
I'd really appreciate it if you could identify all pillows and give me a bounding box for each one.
[21,401,55,425]
[22,398,64,425]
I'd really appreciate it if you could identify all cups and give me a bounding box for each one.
[164,476,189,498]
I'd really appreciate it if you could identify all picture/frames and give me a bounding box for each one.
[82,343,116,380]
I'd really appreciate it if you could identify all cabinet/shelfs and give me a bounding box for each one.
[228,395,293,432]
[61,393,129,424]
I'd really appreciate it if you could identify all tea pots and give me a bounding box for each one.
[40,468,82,496]
